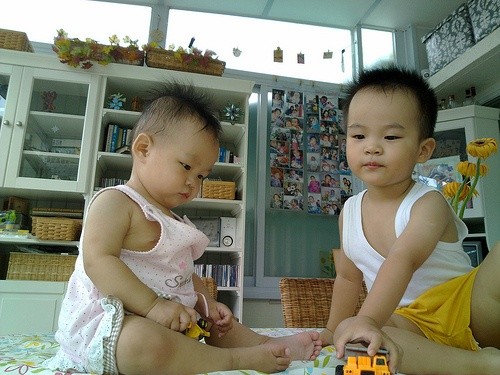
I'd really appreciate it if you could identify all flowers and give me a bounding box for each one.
[53,27,217,69]
[445,138,497,221]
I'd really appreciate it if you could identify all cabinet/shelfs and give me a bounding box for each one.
[0,49,256,335]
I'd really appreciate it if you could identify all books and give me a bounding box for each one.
[104,119,135,154]
[49,138,81,154]
[192,262,241,287]
[94,176,127,192]
[216,146,240,163]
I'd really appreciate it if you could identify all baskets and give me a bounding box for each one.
[146,47,226,76]
[279,276,369,327]
[201,179,237,199]
[68,39,144,66]
[31,216,82,241]
[5,251,78,281]
[0,28,34,52]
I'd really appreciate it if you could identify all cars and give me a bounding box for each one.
[335,342,392,375]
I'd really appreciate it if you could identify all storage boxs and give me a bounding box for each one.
[421,0,500,77]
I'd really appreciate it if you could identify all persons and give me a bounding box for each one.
[290,198,301,209]
[41,76,322,375]
[285,118,302,130]
[270,171,281,187]
[340,177,352,194]
[310,156,318,170]
[319,63,500,375]
[287,170,301,182]
[307,175,321,193]
[272,195,281,208]
[270,107,284,128]
[321,173,337,187]
[323,109,335,120]
[321,147,331,160]
[307,195,318,213]
